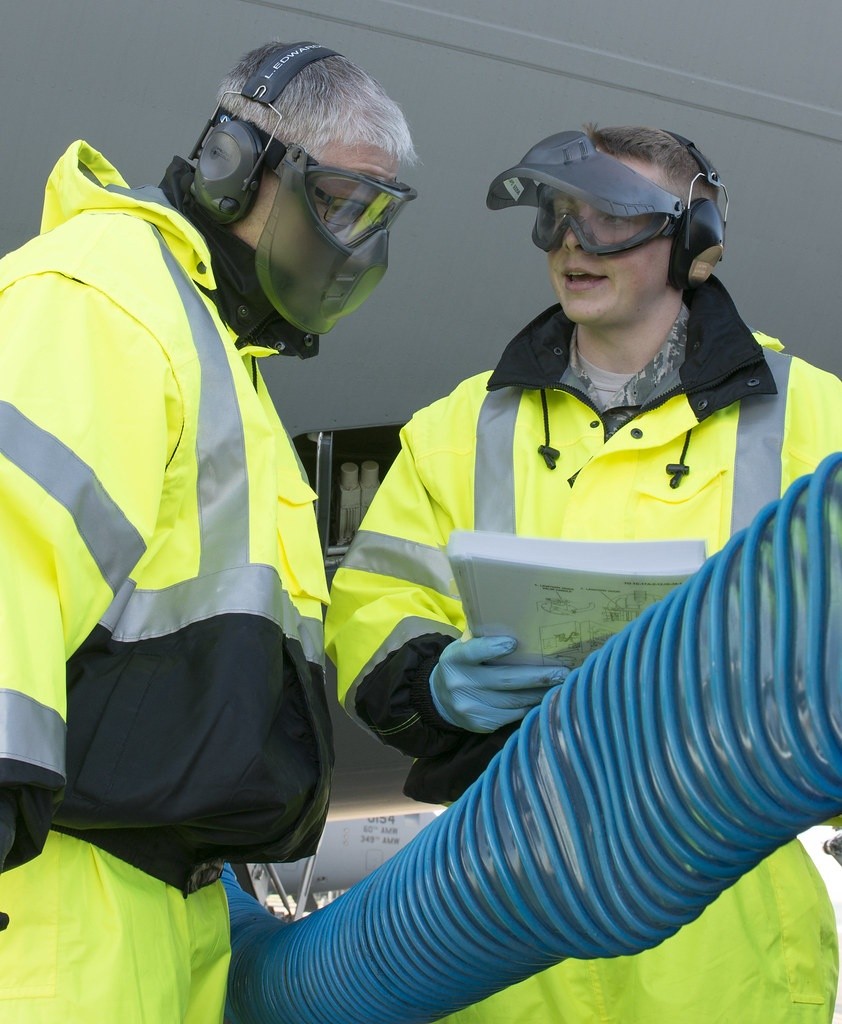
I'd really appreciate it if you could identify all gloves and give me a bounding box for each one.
[428,632,571,736]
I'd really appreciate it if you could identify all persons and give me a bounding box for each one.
[323,125,841,1023]
[0,44,420,1024]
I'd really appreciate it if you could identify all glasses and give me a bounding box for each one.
[313,186,396,231]
[531,176,676,254]
